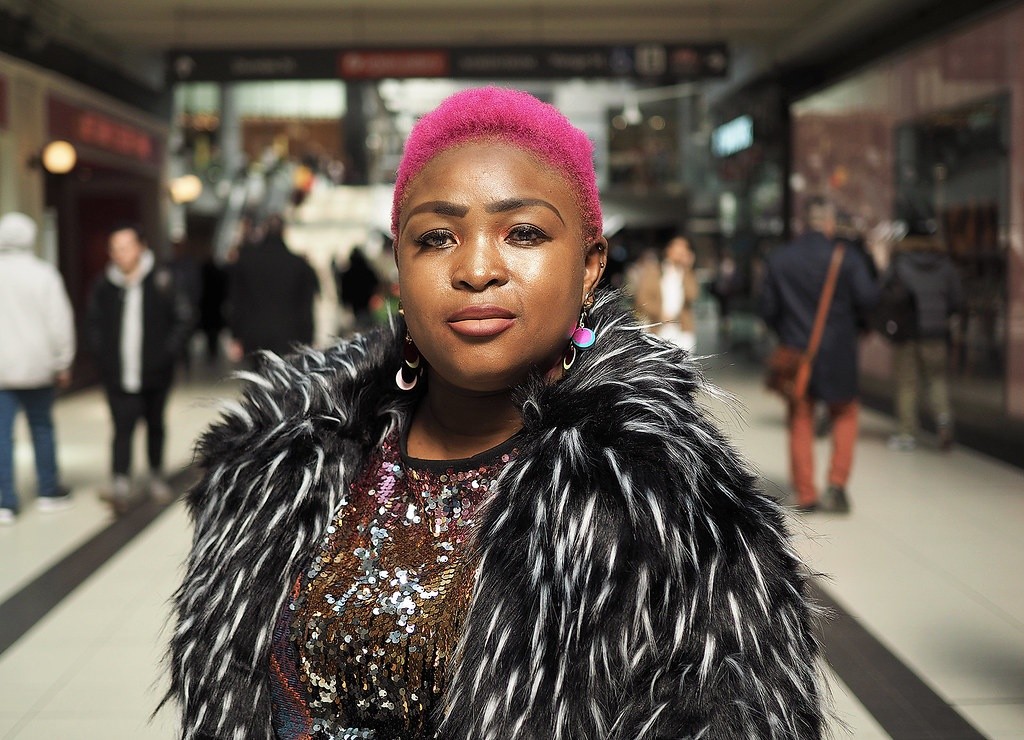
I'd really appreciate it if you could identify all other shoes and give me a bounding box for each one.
[825,485,849,513]
[0,508,14,525]
[34,487,71,511]
[112,477,132,500]
[800,501,823,512]
[148,474,167,500]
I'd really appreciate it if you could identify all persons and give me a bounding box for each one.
[153,207,322,373]
[0,209,78,525]
[337,238,402,332]
[752,193,897,509]
[148,88,838,740]
[877,244,962,452]
[91,228,193,499]
[632,231,701,354]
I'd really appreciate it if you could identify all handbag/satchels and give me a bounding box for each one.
[765,349,811,400]
